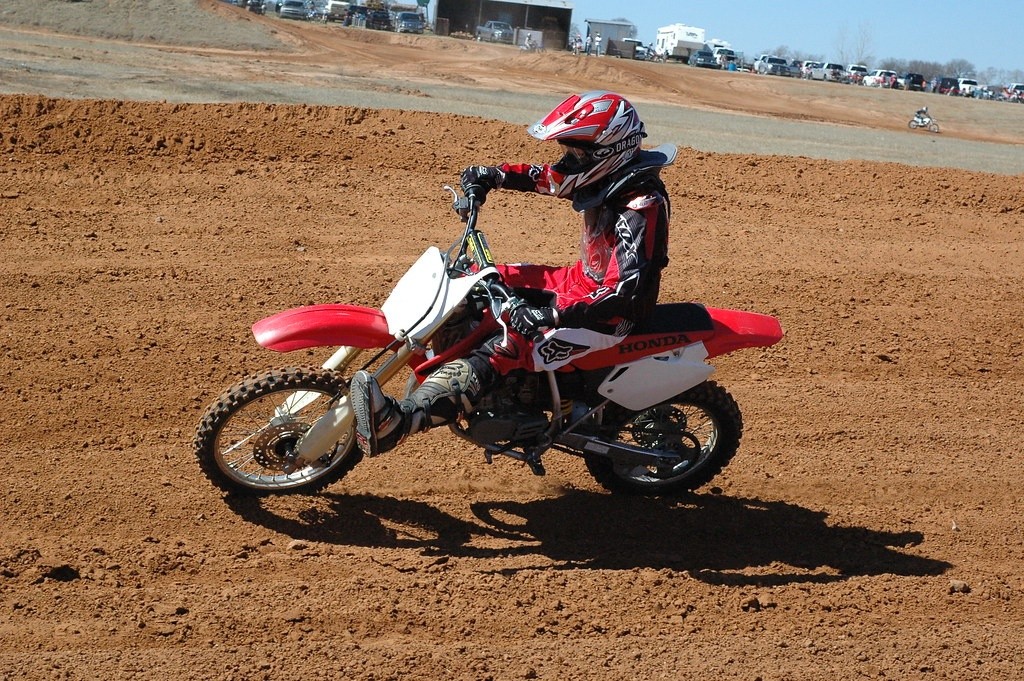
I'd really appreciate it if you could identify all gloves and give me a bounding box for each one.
[509,305,555,340]
[459,165,506,191]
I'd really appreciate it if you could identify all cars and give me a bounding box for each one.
[688,50,719,68]
[930,77,960,95]
[906,74,927,92]
[226,0,393,31]
[896,76,906,87]
[789,58,801,77]
[753,55,778,73]
[957,78,980,99]
[1000,83,1024,100]
[714,48,736,65]
[394,12,424,34]
[862,69,898,87]
[808,62,845,82]
[845,64,868,78]
[802,60,821,78]
[758,56,790,77]
[621,37,647,60]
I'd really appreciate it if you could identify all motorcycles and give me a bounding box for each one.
[643,49,668,65]
[518,39,546,54]
[908,114,940,133]
[192,188,785,497]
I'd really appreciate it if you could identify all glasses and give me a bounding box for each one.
[556,140,601,168]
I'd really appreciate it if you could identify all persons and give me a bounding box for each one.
[915,106,928,126]
[247,0,253,12]
[572,33,602,57]
[304,0,375,29]
[526,32,532,53]
[944,81,1024,104]
[647,43,669,63]
[350,91,671,459]
[262,3,267,15]
[831,68,938,93]
[797,64,813,80]
[719,53,756,74]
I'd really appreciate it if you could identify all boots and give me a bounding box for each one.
[351,358,486,458]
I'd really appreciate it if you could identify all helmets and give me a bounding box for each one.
[923,106,927,111]
[527,91,643,198]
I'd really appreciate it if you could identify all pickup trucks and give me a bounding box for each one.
[475,20,514,44]
